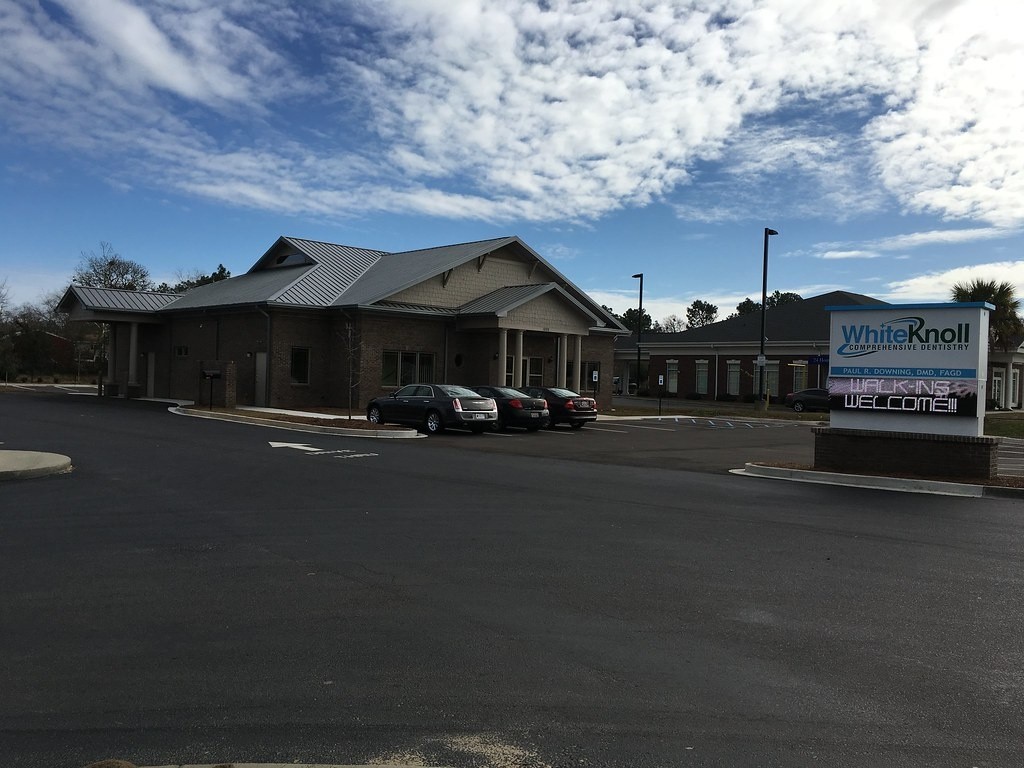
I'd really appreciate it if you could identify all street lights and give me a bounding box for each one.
[755,227,779,401]
[632,273,644,388]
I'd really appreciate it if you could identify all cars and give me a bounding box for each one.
[469,385,552,432]
[364,383,498,436]
[517,386,598,430]
[784,387,831,413]
